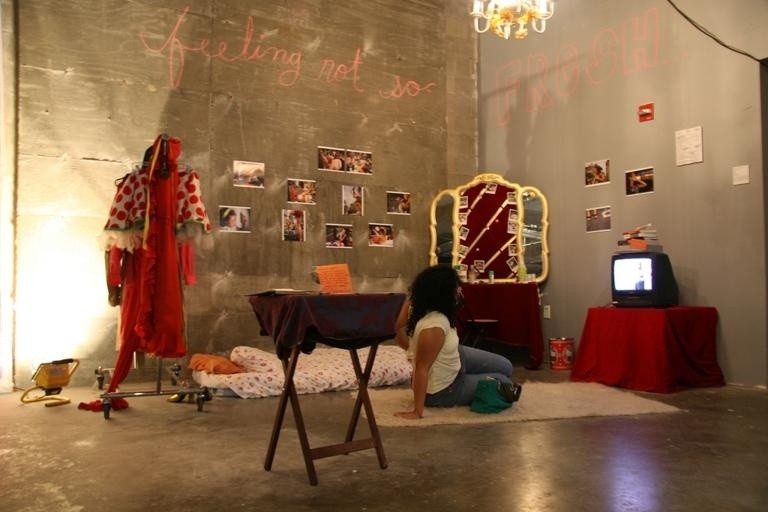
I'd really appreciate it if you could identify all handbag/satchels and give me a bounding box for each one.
[470,379,522,413]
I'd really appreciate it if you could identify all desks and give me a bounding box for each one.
[570,306,726,394]
[247,292,407,486]
[456,281,544,370]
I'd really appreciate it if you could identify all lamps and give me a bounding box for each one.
[22,359,81,406]
[469,0,554,40]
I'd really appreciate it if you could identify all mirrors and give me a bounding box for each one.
[428,174,550,285]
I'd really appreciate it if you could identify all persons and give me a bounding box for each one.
[593,165,605,183]
[392,264,513,419]
[337,228,347,246]
[226,211,249,231]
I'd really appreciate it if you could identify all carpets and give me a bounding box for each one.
[351,381,690,428]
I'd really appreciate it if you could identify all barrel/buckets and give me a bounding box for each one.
[547,336,576,371]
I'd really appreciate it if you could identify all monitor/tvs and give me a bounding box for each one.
[611,252,679,308]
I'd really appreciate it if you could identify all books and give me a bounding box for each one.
[614,229,663,253]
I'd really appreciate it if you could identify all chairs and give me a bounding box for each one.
[456,281,502,352]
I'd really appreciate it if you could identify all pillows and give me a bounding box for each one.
[187,353,246,375]
[230,345,286,380]
